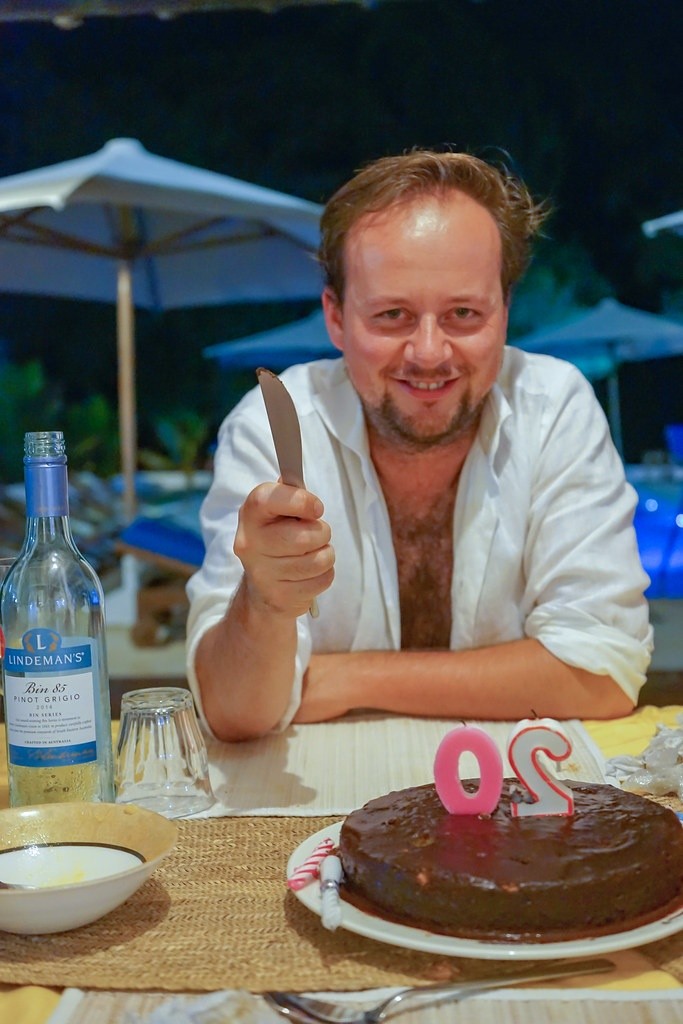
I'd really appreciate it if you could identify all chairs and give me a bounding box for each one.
[111,515,206,648]
[0,468,180,581]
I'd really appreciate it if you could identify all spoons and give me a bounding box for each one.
[263,959,616,1024]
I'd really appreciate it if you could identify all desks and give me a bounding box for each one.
[0,704,683,1024]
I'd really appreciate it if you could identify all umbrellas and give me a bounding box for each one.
[0,133,330,622]
[199,300,348,371]
[505,300,683,461]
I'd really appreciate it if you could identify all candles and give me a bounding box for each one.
[432,708,576,821]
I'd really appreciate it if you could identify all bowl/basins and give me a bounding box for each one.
[0,801,178,933]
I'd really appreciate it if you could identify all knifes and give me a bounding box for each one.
[256,367,306,489]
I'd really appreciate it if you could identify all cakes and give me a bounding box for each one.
[339,775,683,944]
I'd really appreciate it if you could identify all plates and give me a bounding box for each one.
[288,821,683,960]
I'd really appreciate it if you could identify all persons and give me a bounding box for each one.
[184,149,655,745]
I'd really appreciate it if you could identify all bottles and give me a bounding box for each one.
[0,432,114,809]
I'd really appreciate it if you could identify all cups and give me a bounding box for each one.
[116,688,215,818]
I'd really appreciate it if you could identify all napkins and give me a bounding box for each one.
[139,709,623,817]
